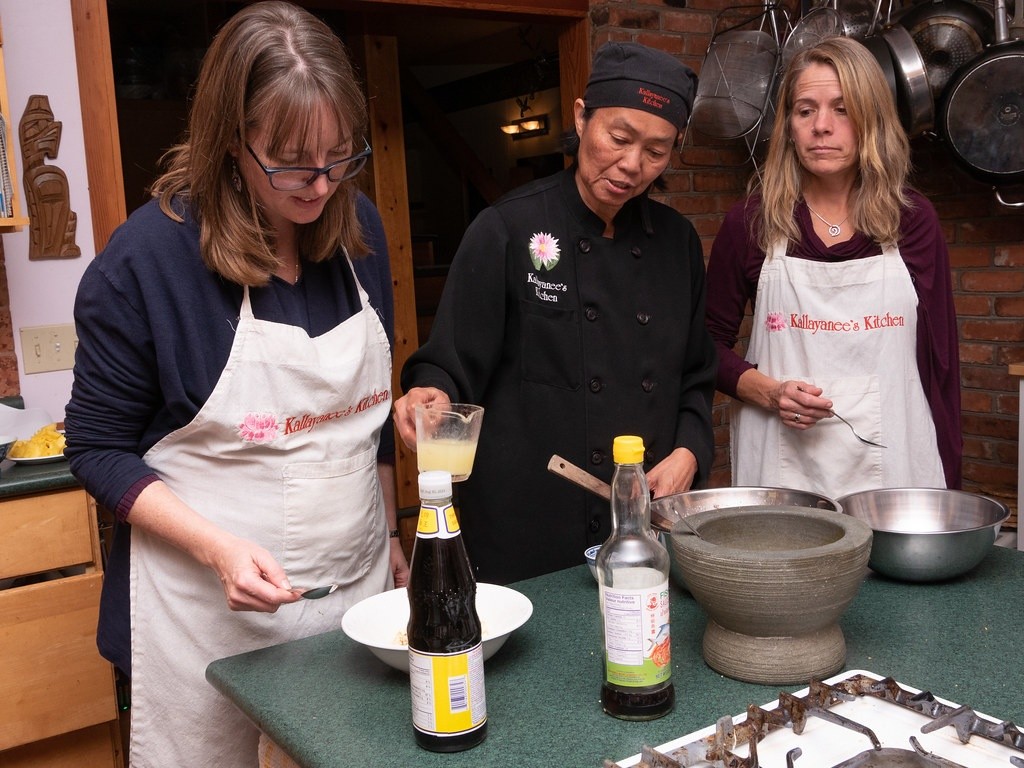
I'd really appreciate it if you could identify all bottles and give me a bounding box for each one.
[597,436,674,721]
[406,471,488,753]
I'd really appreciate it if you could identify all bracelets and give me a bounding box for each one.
[388,529,399,538]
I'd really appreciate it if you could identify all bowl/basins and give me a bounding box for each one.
[835,487,1010,582]
[0,436,18,462]
[650,485,843,590]
[341,582,534,675]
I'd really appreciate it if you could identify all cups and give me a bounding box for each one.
[584,544,606,582]
[414,403,484,482]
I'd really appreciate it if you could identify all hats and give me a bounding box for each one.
[583,40,699,133]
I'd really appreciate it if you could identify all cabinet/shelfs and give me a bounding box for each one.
[0,488,126,768]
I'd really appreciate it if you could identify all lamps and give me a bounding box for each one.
[500,113,549,142]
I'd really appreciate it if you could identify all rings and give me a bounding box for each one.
[795,414,800,423]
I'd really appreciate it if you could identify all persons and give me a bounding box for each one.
[388,40,717,581]
[65,0,411,768]
[694,37,962,504]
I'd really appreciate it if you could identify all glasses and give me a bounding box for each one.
[236,125,373,191]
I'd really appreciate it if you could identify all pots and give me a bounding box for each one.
[899,0,1024,206]
[859,1,933,137]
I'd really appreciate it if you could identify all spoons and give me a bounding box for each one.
[288,585,339,599]
[797,385,887,450]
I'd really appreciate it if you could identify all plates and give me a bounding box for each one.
[6,455,64,464]
